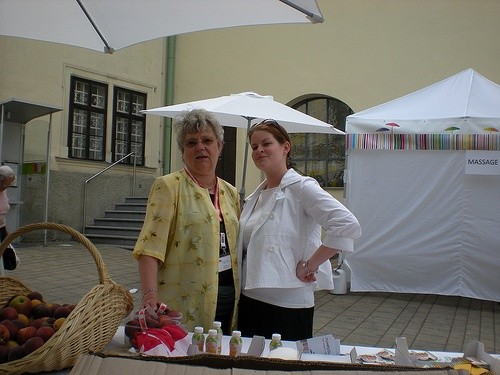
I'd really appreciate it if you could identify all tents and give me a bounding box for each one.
[340,68,500,302]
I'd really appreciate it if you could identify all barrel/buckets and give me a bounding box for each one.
[329,267,347,294]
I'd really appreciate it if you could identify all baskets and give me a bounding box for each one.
[0,222,134,374]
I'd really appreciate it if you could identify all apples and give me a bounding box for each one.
[125,310,180,346]
[0,290,76,365]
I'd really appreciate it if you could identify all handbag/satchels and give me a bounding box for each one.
[3,242,20,270]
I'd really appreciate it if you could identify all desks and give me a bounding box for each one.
[101,326,500,366]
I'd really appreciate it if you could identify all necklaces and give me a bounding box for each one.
[193,176,216,190]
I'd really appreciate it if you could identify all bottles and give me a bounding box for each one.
[228,330,243,357]
[269,334,283,351]
[212,321,223,354]
[205,329,219,354]
[191,326,205,354]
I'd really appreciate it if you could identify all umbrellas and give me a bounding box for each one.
[0,0,324,54]
[140,92,347,209]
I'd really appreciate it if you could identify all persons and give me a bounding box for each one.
[237,121,362,341]
[0,166,16,277]
[133,109,241,335]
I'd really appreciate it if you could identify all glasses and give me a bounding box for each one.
[250,119,286,136]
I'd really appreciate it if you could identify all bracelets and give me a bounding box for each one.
[142,288,158,299]
[303,259,319,275]
